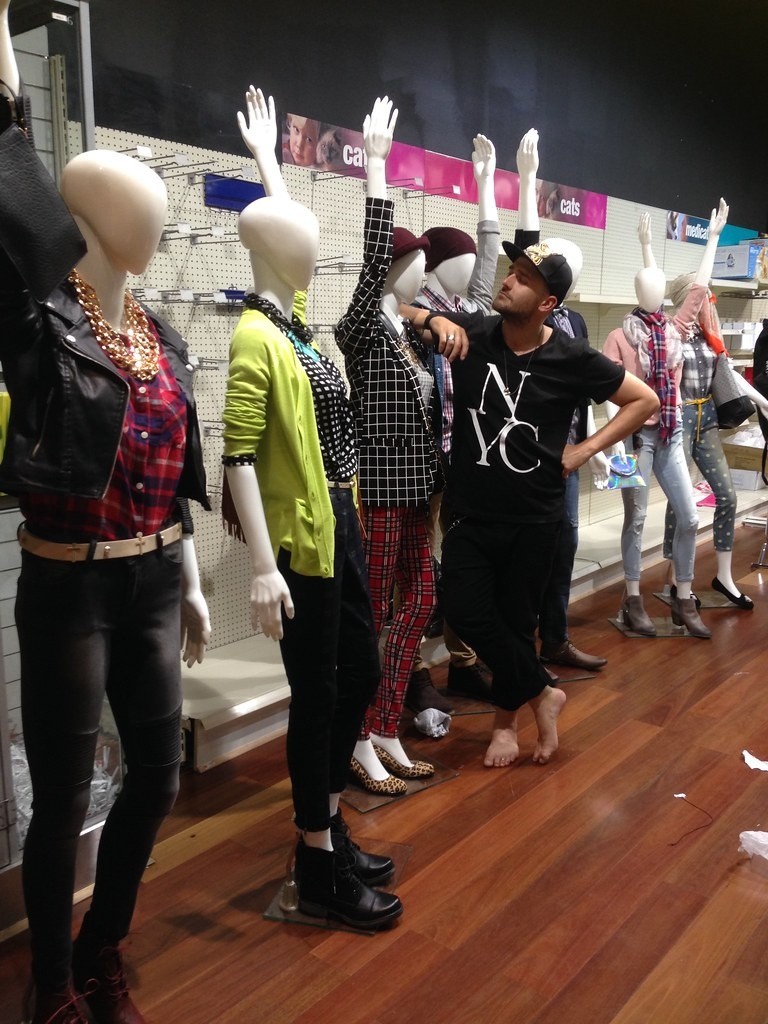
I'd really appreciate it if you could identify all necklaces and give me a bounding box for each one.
[504,321,543,396]
[69,270,162,382]
[377,309,419,362]
[243,294,313,345]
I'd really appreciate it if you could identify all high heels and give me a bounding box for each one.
[671,598,712,637]
[622,588,656,635]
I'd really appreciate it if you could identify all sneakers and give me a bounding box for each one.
[447,661,495,701]
[404,667,455,717]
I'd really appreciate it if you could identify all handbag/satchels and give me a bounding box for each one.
[711,352,756,429]
[0,99,89,304]
[606,454,647,489]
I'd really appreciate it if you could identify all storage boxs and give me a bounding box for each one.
[720,322,763,386]
[710,237,768,290]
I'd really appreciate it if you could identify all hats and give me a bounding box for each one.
[392,227,431,263]
[422,227,477,272]
[502,240,573,309]
[670,274,695,309]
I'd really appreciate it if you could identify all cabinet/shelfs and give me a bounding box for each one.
[707,280,768,360]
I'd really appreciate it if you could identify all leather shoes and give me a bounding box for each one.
[540,639,607,668]
[70,938,146,1024]
[32,977,89,1024]
[545,669,559,681]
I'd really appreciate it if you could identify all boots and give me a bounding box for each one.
[329,807,396,886]
[295,835,403,928]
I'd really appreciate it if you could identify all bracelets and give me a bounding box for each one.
[424,313,444,329]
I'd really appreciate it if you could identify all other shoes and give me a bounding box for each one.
[371,741,435,778]
[349,756,407,795]
[670,584,701,607]
[712,576,754,609]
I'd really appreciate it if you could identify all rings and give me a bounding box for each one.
[446,335,456,341]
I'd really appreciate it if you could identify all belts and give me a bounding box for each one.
[327,481,355,488]
[17,522,183,561]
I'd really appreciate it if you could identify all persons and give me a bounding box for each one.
[603,196,731,638]
[223,84,403,923]
[334,95,435,794]
[515,128,613,680]
[1,0,212,1024]
[286,113,323,167]
[399,237,661,767]
[413,132,500,715]
[637,211,768,613]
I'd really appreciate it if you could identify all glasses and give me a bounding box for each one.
[633,426,643,450]
